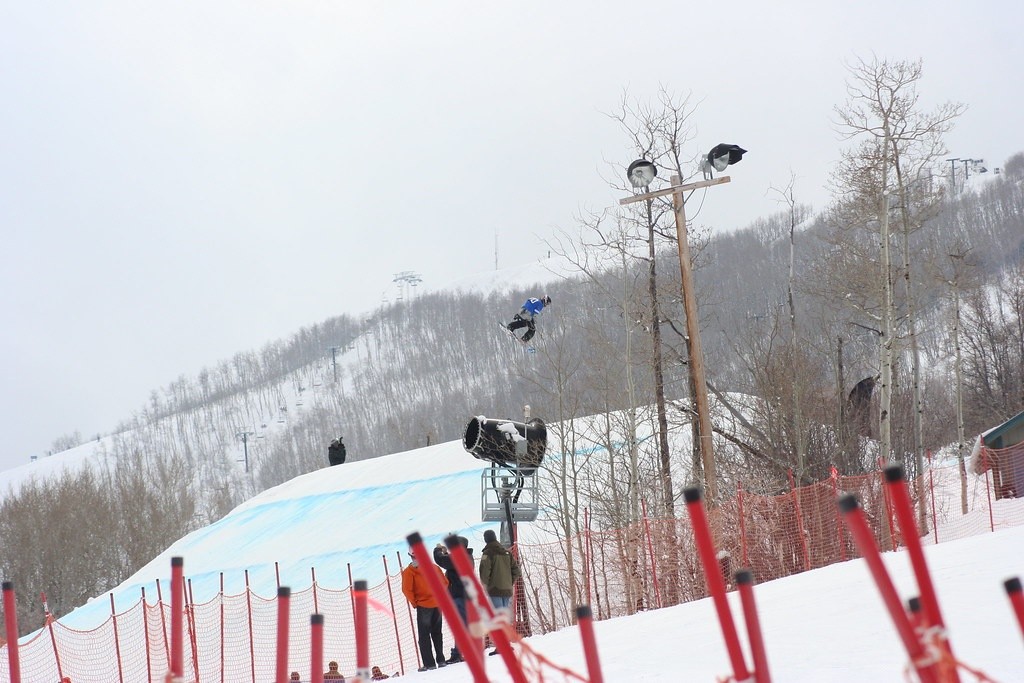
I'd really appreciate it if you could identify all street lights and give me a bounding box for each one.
[619,142,748,553]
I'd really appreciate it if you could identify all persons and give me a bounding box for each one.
[433,537,475,663]
[370,666,389,681]
[327,438,346,466]
[479,531,520,657]
[402,548,449,672]
[445,647,465,664]
[290,671,301,683]
[508,296,552,342]
[323,661,345,683]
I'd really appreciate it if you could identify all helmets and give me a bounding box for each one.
[330,438,339,446]
[541,294,553,309]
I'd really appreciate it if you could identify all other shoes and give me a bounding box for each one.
[445,655,464,664]
[488,646,500,656]
[436,662,447,667]
[418,665,437,672]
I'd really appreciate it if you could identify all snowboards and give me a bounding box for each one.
[498,322,536,356]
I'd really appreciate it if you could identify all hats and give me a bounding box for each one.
[484,530,497,542]
[407,546,415,557]
[458,537,469,546]
